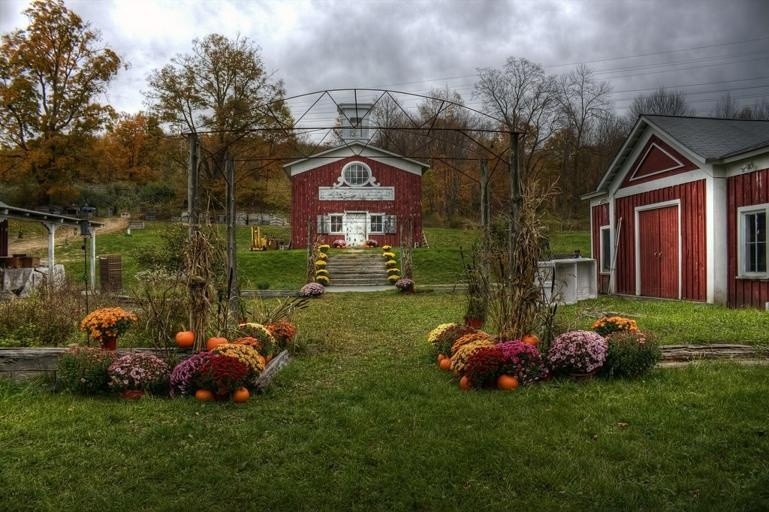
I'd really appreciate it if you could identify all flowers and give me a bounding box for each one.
[333,239,346,246]
[78,305,296,397]
[366,240,377,245]
[382,244,415,291]
[425,314,644,390]
[299,244,330,296]
